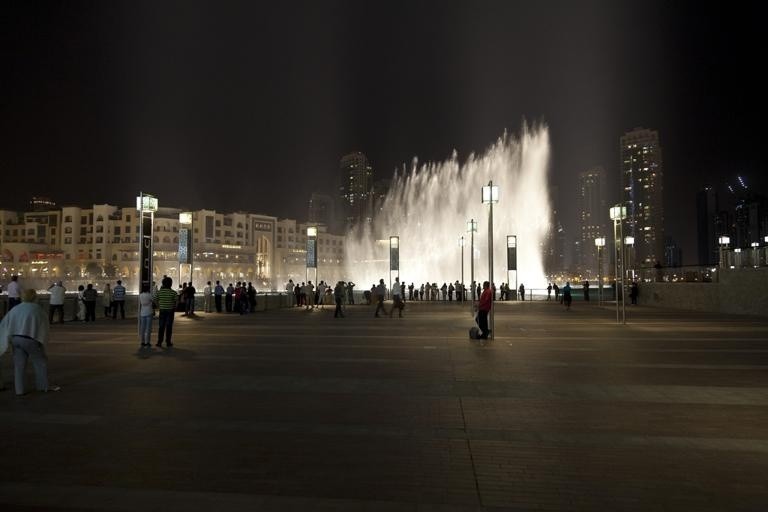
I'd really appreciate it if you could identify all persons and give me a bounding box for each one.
[654,261,664,282]
[2,289,61,397]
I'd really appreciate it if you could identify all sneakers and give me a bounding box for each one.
[140,341,174,349]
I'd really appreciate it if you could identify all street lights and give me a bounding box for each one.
[610,201,629,327]
[594,236,605,307]
[506,234,518,302]
[135,191,159,294]
[177,211,195,289]
[304,226,318,294]
[623,234,635,283]
[389,235,399,301]
[718,234,768,271]
[457,232,464,306]
[481,180,498,343]
[466,218,478,317]
